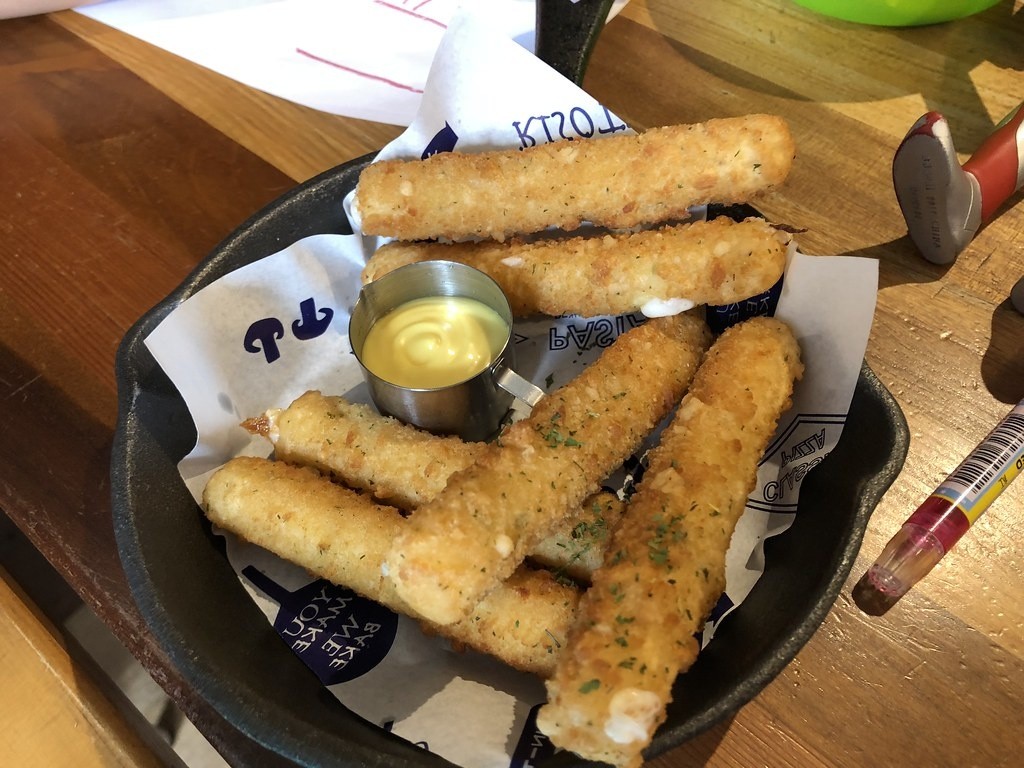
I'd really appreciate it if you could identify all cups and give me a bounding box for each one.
[350,260,546,442]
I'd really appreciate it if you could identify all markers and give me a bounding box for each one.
[869,399,1024,607]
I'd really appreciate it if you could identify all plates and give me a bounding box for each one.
[111,0,911,768]
